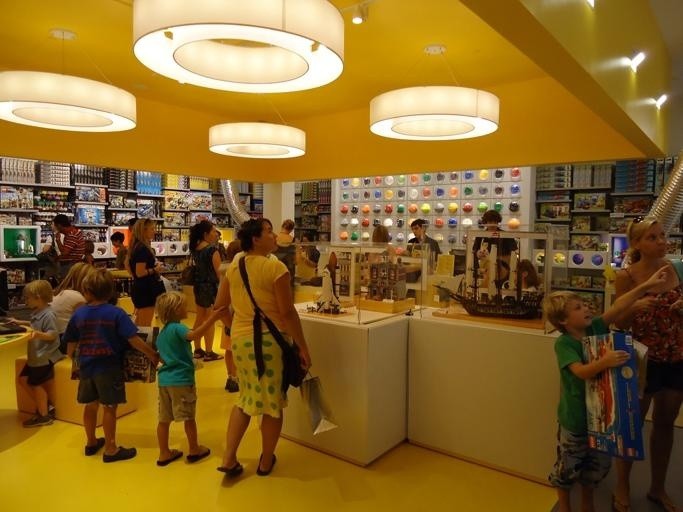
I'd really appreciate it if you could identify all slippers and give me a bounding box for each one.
[647,492,678,511]
[157,450,182,466]
[187,445,210,463]
[216,462,243,473]
[611,495,631,511]
[256,453,276,476]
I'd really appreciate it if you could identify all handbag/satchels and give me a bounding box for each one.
[128,279,164,309]
[281,343,306,388]
[182,266,194,286]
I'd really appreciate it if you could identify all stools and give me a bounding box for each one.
[16,351,138,426]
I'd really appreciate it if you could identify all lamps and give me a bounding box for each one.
[352,5,363,24]
[0,29,136,132]
[369,44,500,140]
[208,94,306,158]
[132,0,345,93]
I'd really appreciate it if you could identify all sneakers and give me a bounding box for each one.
[22,403,55,428]
[85,437,104,455]
[104,447,136,462]
[225,379,239,392]
[203,351,224,361]
[194,348,205,358]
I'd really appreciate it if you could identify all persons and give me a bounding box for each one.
[299,233,321,282]
[154,291,230,467]
[53,213,86,279]
[514,260,537,288]
[217,240,243,394]
[48,261,100,355]
[367,226,396,265]
[213,230,227,261]
[188,220,227,361]
[272,220,298,302]
[42,219,65,257]
[212,217,315,476]
[110,231,128,270]
[613,216,683,512]
[544,291,631,512]
[65,268,160,464]
[406,220,440,262]
[472,211,518,267]
[5,279,63,428]
[81,238,95,266]
[128,218,163,327]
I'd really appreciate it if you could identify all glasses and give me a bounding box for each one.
[632,216,644,224]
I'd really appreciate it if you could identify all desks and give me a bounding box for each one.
[0,319,32,410]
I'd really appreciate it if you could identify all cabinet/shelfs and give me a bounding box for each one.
[533,158,683,317]
